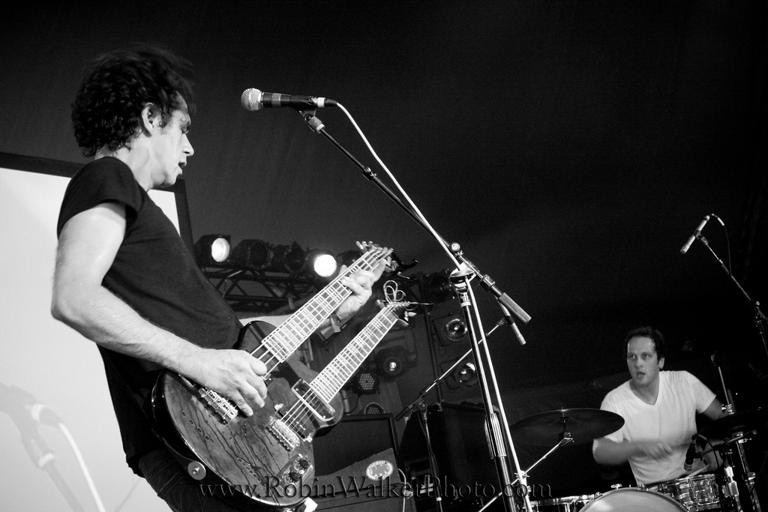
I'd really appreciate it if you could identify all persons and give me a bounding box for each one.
[52,41,373,512]
[592,327,739,490]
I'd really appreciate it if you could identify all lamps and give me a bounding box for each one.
[193,233,232,267]
[307,248,338,277]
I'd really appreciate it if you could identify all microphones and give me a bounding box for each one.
[680,218,710,254]
[239,87,326,112]
[683,443,696,472]
[710,349,719,361]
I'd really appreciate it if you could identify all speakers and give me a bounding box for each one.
[311,413,404,474]
[398,401,497,479]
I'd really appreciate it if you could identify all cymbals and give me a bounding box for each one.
[706,409,768,440]
[509,408,625,445]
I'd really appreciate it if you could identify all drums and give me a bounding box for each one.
[577,486,689,512]
[660,473,721,512]
[524,493,595,512]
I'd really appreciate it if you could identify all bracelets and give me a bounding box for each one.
[330,311,348,331]
